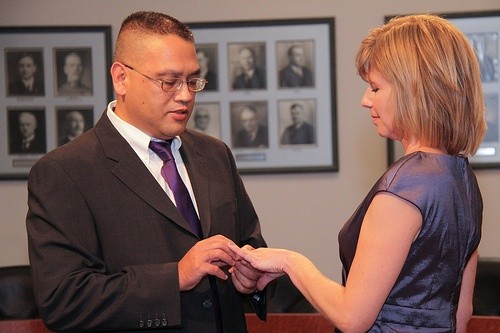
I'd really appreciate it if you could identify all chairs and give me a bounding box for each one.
[0,261,500,320]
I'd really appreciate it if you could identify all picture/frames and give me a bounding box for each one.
[0,24,114,181]
[384,9,500,171]
[184,17,338,175]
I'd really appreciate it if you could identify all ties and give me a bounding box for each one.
[149,138,203,240]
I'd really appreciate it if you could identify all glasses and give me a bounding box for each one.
[123,62,208,92]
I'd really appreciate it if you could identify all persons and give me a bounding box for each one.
[189,106,217,137]
[229,17,482,333]
[279,46,313,85]
[14,112,47,154]
[58,112,87,146]
[282,103,315,144]
[233,46,265,87]
[10,53,44,95]
[58,52,91,96]
[28,11,272,333]
[193,50,217,90]
[234,106,267,146]
